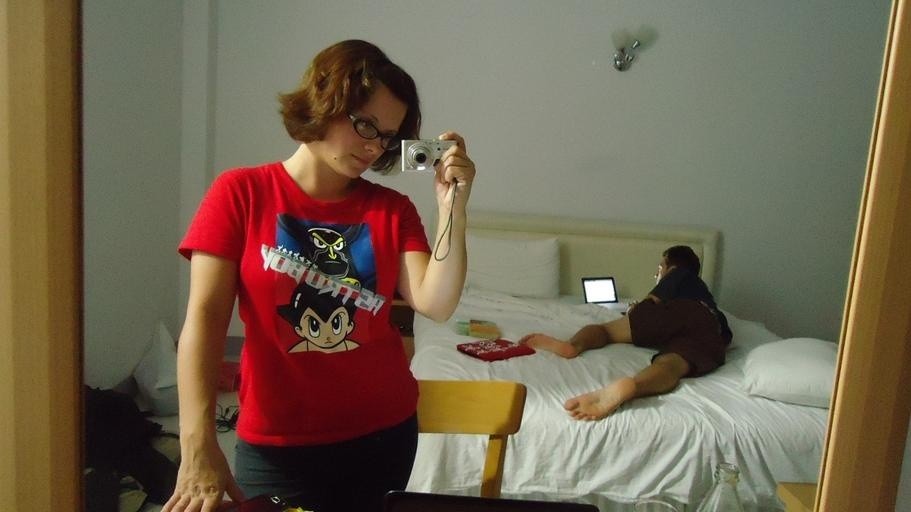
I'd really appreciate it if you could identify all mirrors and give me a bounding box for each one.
[0,0,910,512]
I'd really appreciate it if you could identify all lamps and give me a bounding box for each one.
[610,19,659,72]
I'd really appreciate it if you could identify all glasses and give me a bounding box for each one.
[346,111,401,152]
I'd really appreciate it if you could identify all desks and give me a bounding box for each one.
[774,482,819,512]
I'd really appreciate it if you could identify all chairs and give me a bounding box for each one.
[414,381,528,499]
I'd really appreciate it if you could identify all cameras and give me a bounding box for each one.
[400,136,460,174]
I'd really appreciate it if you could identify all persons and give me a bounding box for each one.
[160,37,479,512]
[516,245,735,422]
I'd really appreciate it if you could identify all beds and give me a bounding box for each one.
[403,211,839,512]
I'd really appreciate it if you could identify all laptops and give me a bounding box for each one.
[581,275,630,315]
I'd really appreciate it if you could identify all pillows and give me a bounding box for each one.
[437,232,562,297]
[743,339,842,406]
